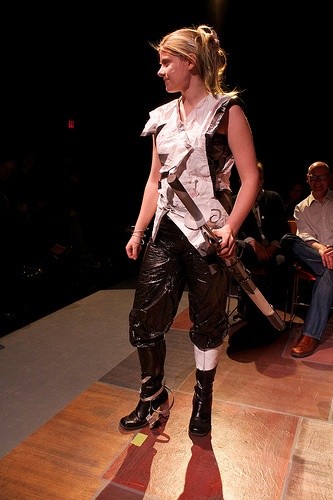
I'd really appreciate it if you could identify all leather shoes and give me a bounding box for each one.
[290,335,319,358]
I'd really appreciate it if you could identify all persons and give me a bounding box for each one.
[121,25,262,435]
[0,155,44,215]
[287,181,307,206]
[232,163,288,323]
[291,161,333,358]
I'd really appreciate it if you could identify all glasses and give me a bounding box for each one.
[1,167,16,177]
[309,175,329,179]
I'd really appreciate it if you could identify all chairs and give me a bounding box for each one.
[287,219,333,334]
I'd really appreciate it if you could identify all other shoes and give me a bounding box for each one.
[0,312,18,323]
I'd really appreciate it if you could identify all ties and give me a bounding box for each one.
[252,202,261,227]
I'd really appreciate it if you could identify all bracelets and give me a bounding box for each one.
[130,226,150,245]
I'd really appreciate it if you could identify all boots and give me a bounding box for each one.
[119,372,169,430]
[189,369,213,436]
